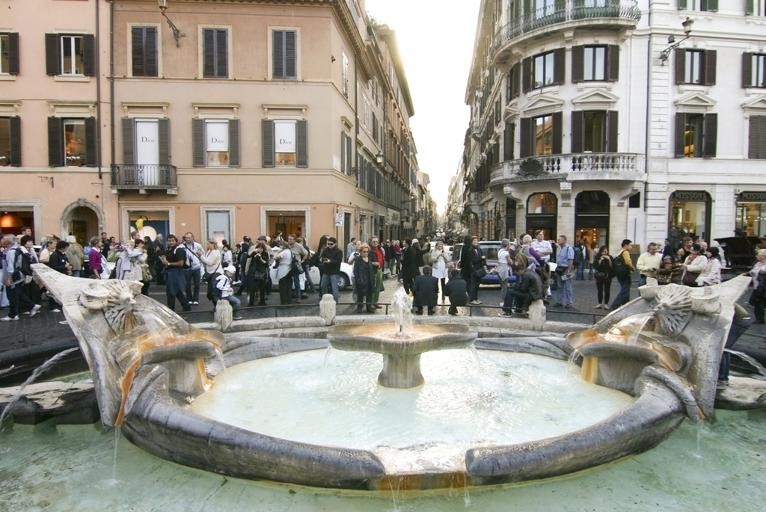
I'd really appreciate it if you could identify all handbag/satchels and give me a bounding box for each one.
[202,273,212,281]
[474,268,486,278]
[613,256,626,276]
[682,272,700,286]
[555,267,566,274]
[292,260,299,275]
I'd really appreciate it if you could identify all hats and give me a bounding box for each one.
[224,266,236,273]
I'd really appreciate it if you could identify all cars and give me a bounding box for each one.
[452,240,560,291]
[241,250,359,292]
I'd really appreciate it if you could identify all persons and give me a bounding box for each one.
[1,225,343,320]
[346,229,486,315]
[636,227,726,297]
[592,239,635,312]
[496,229,575,317]
[734,229,744,237]
[574,238,600,280]
[746,241,766,324]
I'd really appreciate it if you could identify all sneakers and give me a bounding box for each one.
[1,304,69,325]
[416,299,525,317]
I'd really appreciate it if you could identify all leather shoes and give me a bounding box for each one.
[551,303,610,310]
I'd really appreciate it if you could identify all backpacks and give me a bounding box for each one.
[207,278,221,299]
[186,251,192,269]
[12,248,37,276]
